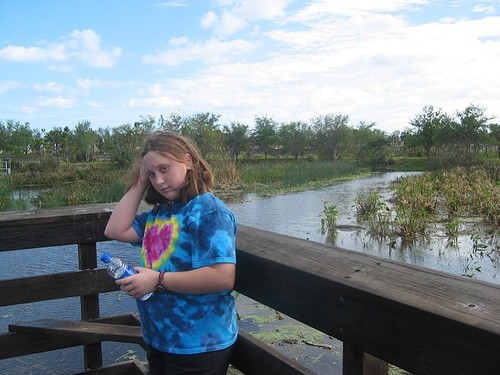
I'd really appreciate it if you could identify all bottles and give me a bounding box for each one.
[101,253,153,300]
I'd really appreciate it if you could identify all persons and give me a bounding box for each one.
[104,132,239,375]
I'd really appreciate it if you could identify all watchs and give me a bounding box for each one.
[156,271,166,294]
